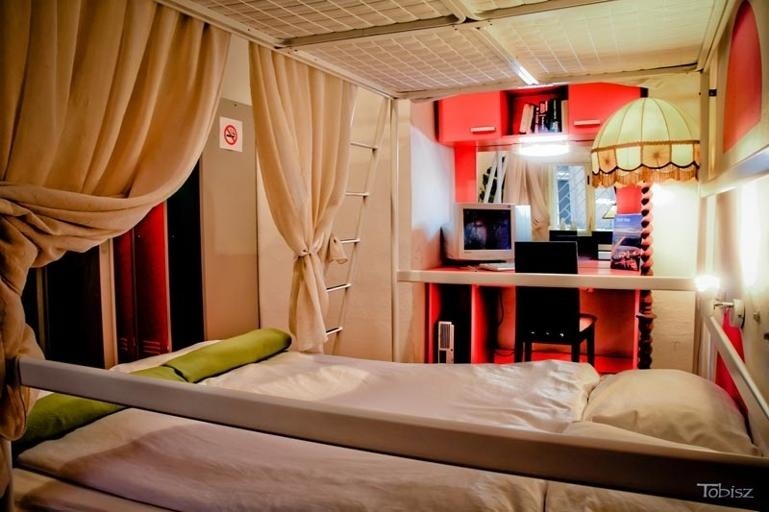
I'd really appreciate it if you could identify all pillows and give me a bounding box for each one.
[544,419,769,512]
[582,369,763,457]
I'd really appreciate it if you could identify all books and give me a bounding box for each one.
[479,262,516,273]
[518,98,569,134]
[611,209,642,272]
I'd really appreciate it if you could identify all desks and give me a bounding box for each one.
[423,258,641,374]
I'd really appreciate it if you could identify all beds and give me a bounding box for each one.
[11,340,769,512]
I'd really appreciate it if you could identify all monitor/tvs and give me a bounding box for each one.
[441,202,515,265]
[515,205,532,242]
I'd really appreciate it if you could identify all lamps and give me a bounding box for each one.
[694,273,744,328]
[591,97,701,370]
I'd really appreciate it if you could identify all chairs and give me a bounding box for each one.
[514,241,597,367]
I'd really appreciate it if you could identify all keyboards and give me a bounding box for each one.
[479,263,515,271]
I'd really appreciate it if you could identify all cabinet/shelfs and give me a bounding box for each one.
[437,83,648,151]
